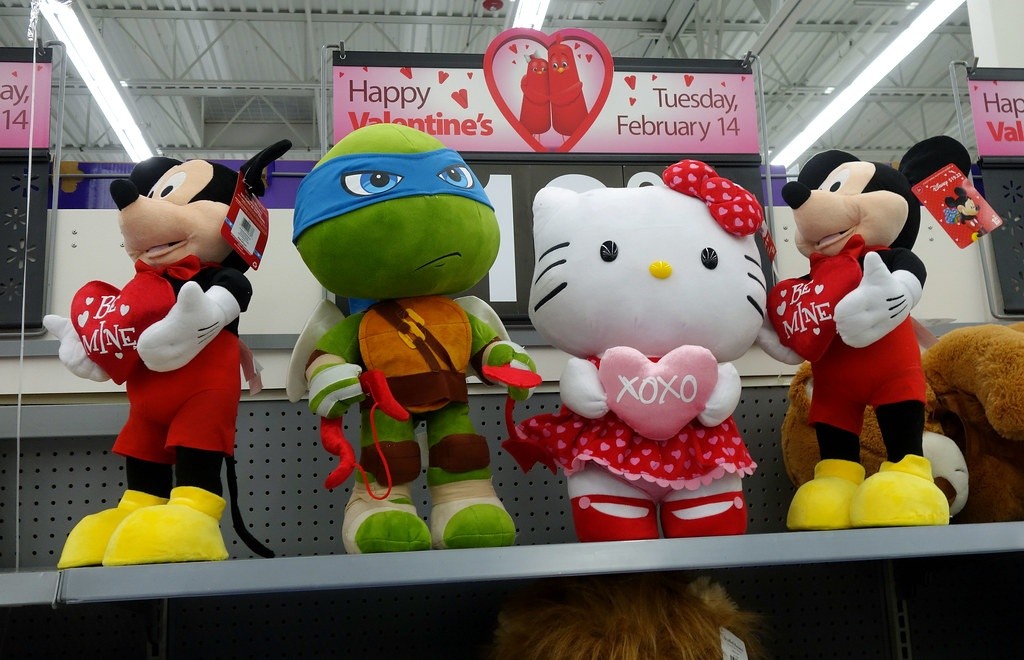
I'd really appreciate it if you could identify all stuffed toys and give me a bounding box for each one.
[494,572,766,658]
[780,324,1023,523]
[762,134,972,527]
[291,123,542,554]
[39,138,295,568]
[516,160,768,541]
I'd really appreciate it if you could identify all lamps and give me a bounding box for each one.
[765,1,967,172]
[508,0,550,34]
[35,1,163,165]
